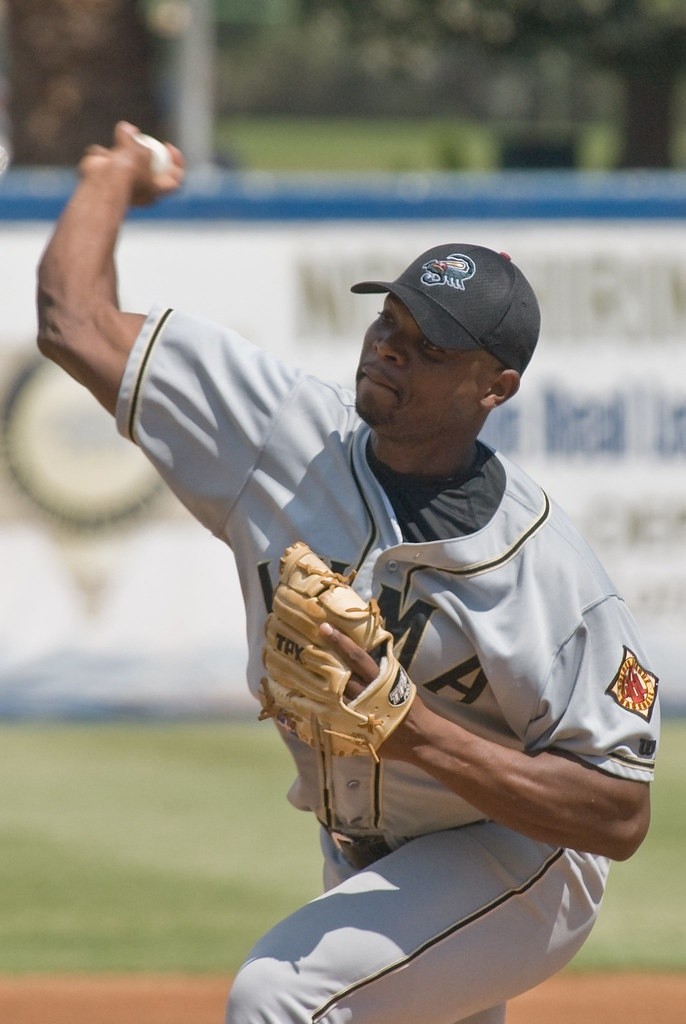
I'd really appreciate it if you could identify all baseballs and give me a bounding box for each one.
[133,131,177,175]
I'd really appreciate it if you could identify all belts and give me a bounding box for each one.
[317,819,391,870]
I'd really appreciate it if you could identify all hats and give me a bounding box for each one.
[350,244,541,378]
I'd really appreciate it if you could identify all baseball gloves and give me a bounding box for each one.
[255,536,422,763]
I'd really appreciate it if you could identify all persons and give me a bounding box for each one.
[37,122,663,1024]
